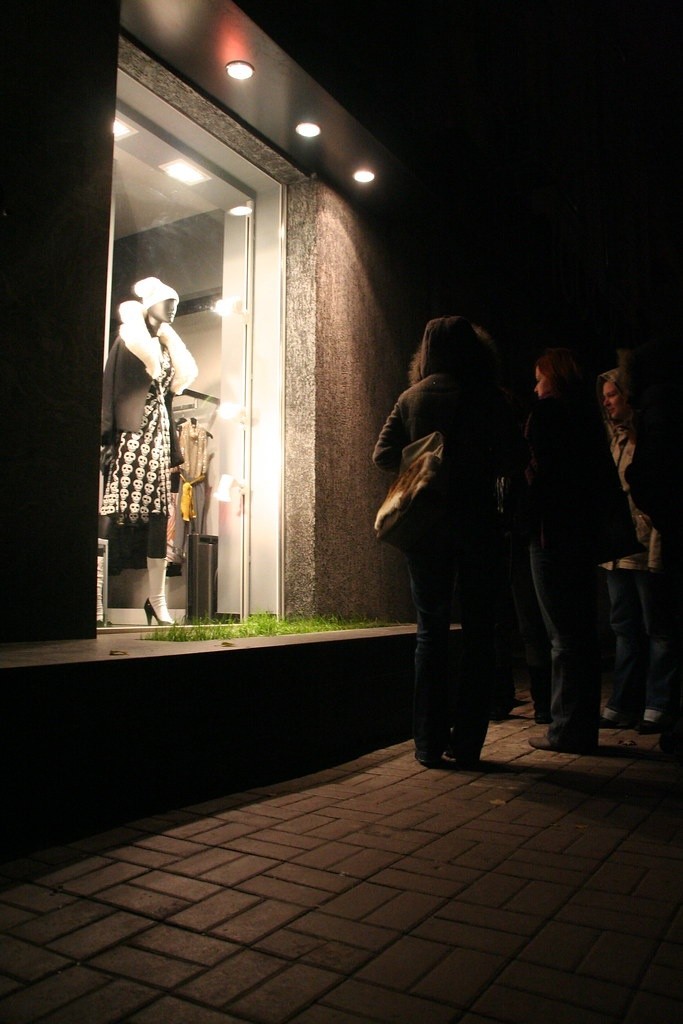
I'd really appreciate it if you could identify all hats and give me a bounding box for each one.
[133,277,179,310]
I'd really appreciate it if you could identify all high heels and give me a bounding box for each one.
[97,613,107,629]
[143,597,176,626]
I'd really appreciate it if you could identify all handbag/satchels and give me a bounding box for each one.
[373,429,448,552]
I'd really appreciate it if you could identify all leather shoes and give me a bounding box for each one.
[489,710,507,719]
[534,711,551,724]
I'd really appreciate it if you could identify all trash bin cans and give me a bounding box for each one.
[188,532,219,623]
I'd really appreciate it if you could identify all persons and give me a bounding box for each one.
[487,347,683,756]
[97,277,198,626]
[373,315,529,768]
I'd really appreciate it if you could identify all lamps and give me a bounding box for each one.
[217,401,261,429]
[213,473,246,504]
[211,294,251,326]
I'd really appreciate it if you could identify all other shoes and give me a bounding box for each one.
[527,737,553,750]
[640,720,663,733]
[441,751,479,767]
[598,715,622,728]
[414,753,440,763]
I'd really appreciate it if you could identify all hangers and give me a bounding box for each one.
[175,402,214,440]
